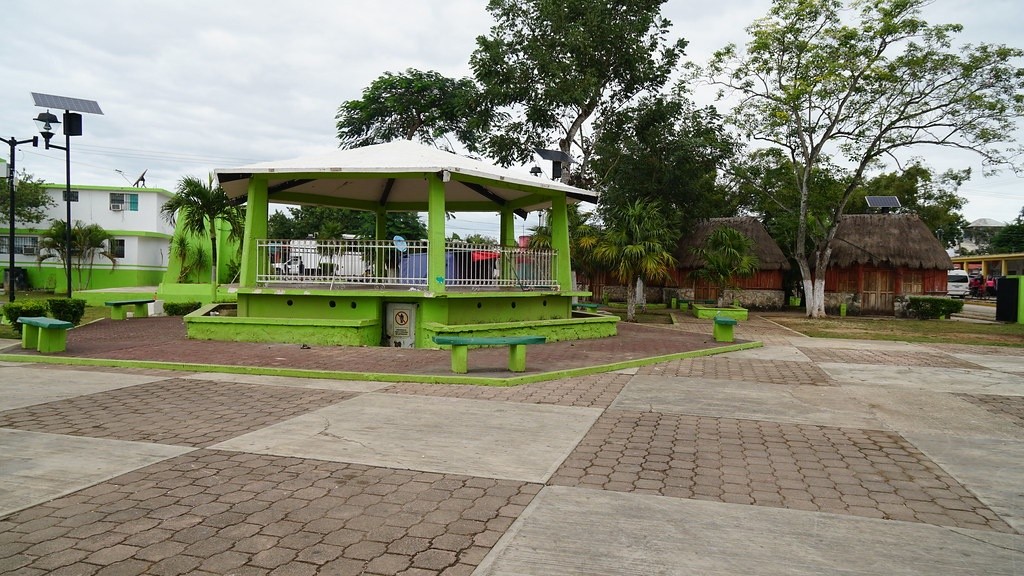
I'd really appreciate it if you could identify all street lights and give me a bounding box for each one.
[32,93,102,296]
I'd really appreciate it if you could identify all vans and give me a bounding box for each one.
[946,268,971,300]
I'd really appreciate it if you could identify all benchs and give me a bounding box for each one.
[105,299,155,320]
[679,300,715,310]
[432,336,546,374]
[17,317,73,353]
[713,316,737,342]
[572,303,598,312]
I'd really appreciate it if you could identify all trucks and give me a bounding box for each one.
[270,251,386,275]
